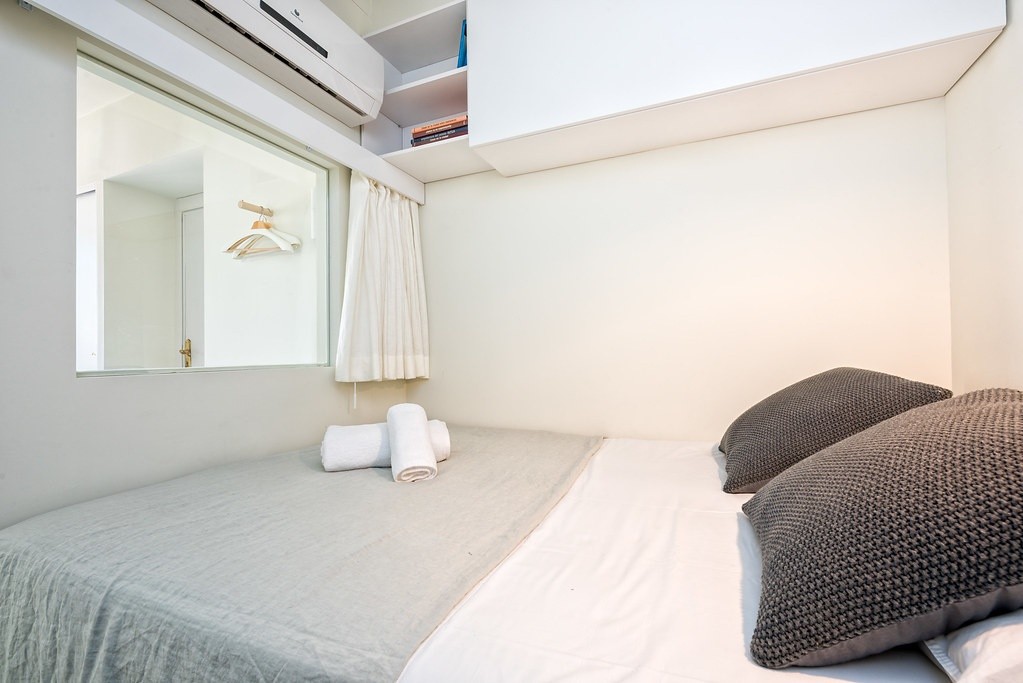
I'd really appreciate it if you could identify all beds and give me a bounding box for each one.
[0,425,953,683]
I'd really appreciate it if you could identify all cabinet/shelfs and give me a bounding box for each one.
[359,0,1006,186]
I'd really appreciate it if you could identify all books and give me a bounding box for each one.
[410,113,468,147]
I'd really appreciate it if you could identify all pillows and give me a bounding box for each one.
[919,607,1023,683]
[717,368,954,493]
[742,389,1023,671]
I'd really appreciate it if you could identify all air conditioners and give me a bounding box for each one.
[145,0,386,128]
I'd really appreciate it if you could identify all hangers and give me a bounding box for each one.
[221,206,302,259]
